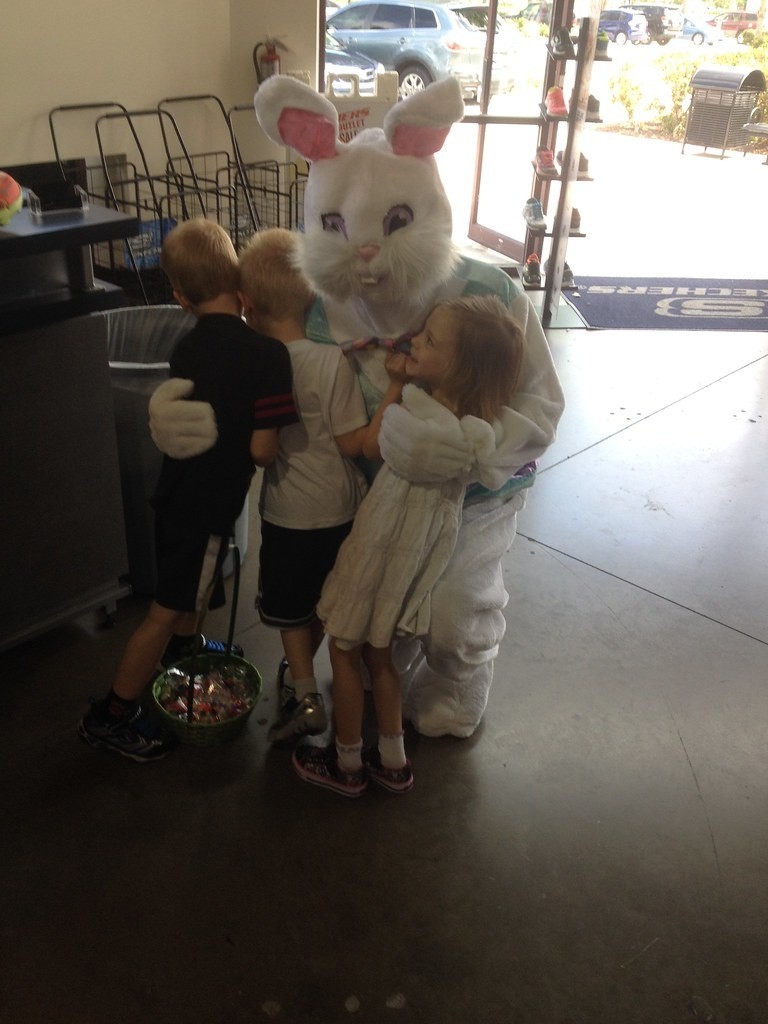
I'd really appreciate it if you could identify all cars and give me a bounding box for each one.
[496,1,761,61]
[323,25,386,98]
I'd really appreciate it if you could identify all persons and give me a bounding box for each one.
[292,295,526,796]
[85,219,298,762]
[238,232,367,742]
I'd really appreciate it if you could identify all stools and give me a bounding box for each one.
[742,104,768,165]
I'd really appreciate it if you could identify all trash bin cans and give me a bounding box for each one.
[680,64,767,161]
[83,306,252,585]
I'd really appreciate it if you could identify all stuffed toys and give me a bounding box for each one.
[145,71,564,740]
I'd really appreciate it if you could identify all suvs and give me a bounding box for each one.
[324,0,525,104]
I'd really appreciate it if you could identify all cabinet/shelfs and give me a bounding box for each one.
[516,42,603,293]
[0,158,136,652]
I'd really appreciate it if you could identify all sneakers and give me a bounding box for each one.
[290,755,416,799]
[166,636,244,670]
[81,703,169,763]
[269,656,327,749]
